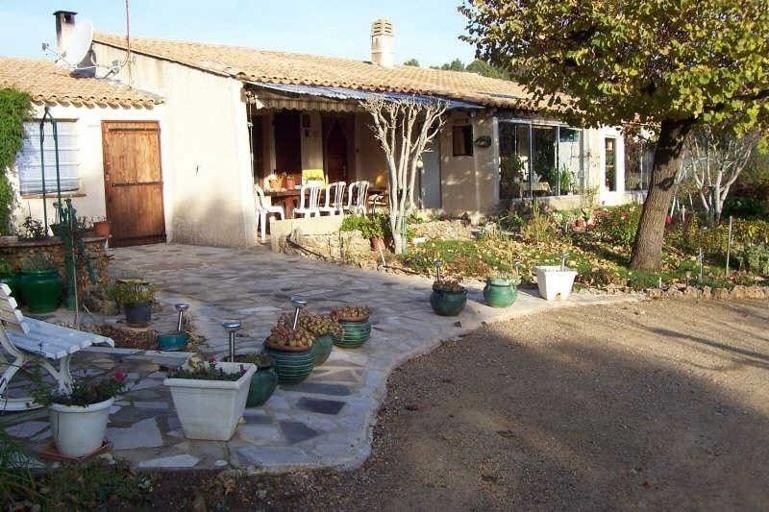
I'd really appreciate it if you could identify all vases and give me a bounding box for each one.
[93,220,113,237]
[535,264,579,302]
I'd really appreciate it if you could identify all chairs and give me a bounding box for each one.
[252,167,389,243]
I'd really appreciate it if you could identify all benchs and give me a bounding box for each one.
[0,283,117,414]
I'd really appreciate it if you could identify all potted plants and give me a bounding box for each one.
[483,265,522,308]
[499,153,525,198]
[429,279,469,317]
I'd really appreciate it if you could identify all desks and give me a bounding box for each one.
[520,181,552,197]
